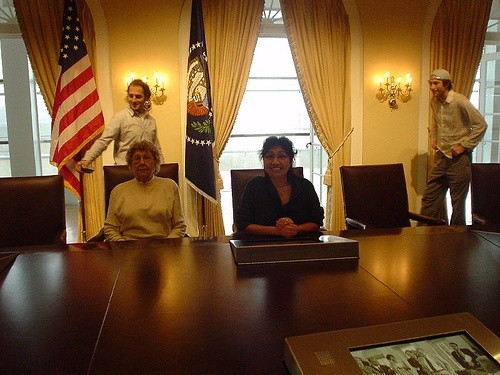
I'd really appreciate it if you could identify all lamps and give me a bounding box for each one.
[124,70,168,106]
[376,70,413,108]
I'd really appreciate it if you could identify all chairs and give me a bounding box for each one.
[471,162,500,225]
[339,162,446,230]
[86,163,192,242]
[231,167,328,233]
[0,175,68,244]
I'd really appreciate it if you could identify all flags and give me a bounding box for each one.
[184,0,218,205]
[50,0,105,199]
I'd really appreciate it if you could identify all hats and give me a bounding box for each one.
[430,68,455,89]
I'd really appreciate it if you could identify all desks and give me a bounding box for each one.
[0,225,500,375]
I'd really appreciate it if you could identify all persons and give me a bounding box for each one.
[406,348,457,375]
[449,342,482,370]
[385,354,413,375]
[356,357,396,375]
[420,69,487,224]
[76,79,164,173]
[236,136,324,238]
[104,140,187,241]
